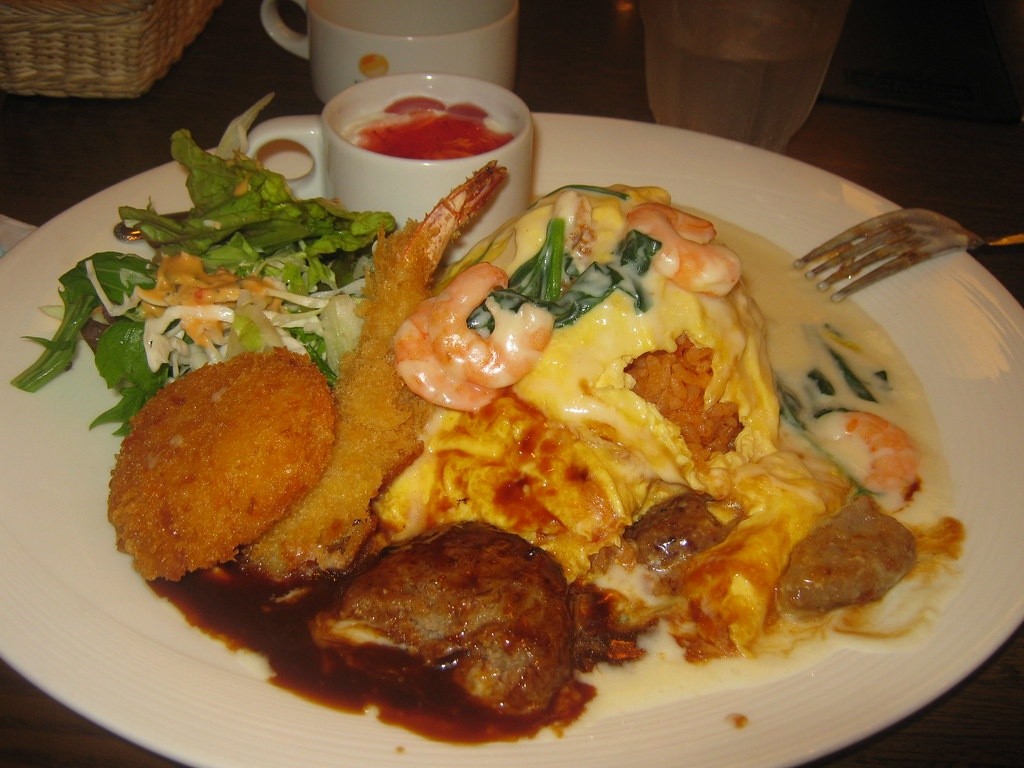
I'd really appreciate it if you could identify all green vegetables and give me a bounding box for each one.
[9,94,891,442]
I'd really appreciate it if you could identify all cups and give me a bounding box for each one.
[637,0,848,153]
[260,0,517,106]
[243,74,533,269]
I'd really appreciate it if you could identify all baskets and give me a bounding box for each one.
[0,0,220,99]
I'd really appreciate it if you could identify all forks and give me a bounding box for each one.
[793,207,1024,304]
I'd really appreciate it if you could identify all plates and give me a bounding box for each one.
[0,114,1024,768]
